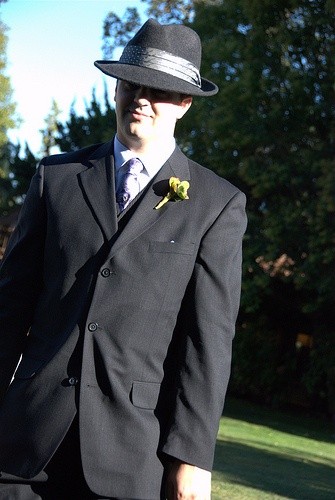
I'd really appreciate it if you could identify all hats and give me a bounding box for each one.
[94,19,219,97]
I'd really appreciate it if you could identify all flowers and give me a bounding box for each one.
[154,176,190,209]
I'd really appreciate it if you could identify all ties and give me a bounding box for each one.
[116,159,143,217]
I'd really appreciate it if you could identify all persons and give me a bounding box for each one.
[1,18,247,500]
